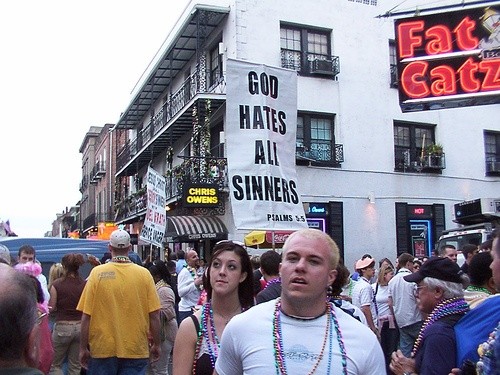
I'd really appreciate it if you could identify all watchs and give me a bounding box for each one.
[402,371,416,375]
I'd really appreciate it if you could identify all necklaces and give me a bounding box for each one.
[214,311,242,326]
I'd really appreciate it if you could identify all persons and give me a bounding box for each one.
[0,263,47,375]
[389,257,500,375]
[11,246,50,305]
[172,240,256,375]
[352,254,381,342]
[440,245,457,262]
[213,229,386,375]
[449,225,500,375]
[22,275,54,375]
[167,261,181,326]
[148,260,178,375]
[388,253,425,358]
[76,229,161,375]
[463,251,496,310]
[371,265,398,375]
[340,268,357,303]
[461,240,492,273]
[249,251,282,305]
[144,250,206,274]
[379,250,438,273]
[327,264,367,326]
[49,254,87,375]
[177,250,203,327]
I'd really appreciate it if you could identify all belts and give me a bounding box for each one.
[55,322,80,326]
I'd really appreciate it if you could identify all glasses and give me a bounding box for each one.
[385,267,392,270]
[23,309,47,332]
[413,285,428,290]
[361,254,372,260]
[216,240,248,253]
[413,266,421,269]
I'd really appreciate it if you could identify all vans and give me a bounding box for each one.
[1,237,142,281]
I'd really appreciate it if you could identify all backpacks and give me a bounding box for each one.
[439,295,500,369]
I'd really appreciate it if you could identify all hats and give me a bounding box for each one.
[356,257,375,269]
[110,229,131,249]
[403,257,464,283]
[0,245,10,264]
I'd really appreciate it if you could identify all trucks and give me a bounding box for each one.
[437,198,500,267]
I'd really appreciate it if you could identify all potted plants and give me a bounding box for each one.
[424,143,443,165]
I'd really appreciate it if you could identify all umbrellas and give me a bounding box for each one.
[244,230,296,255]
